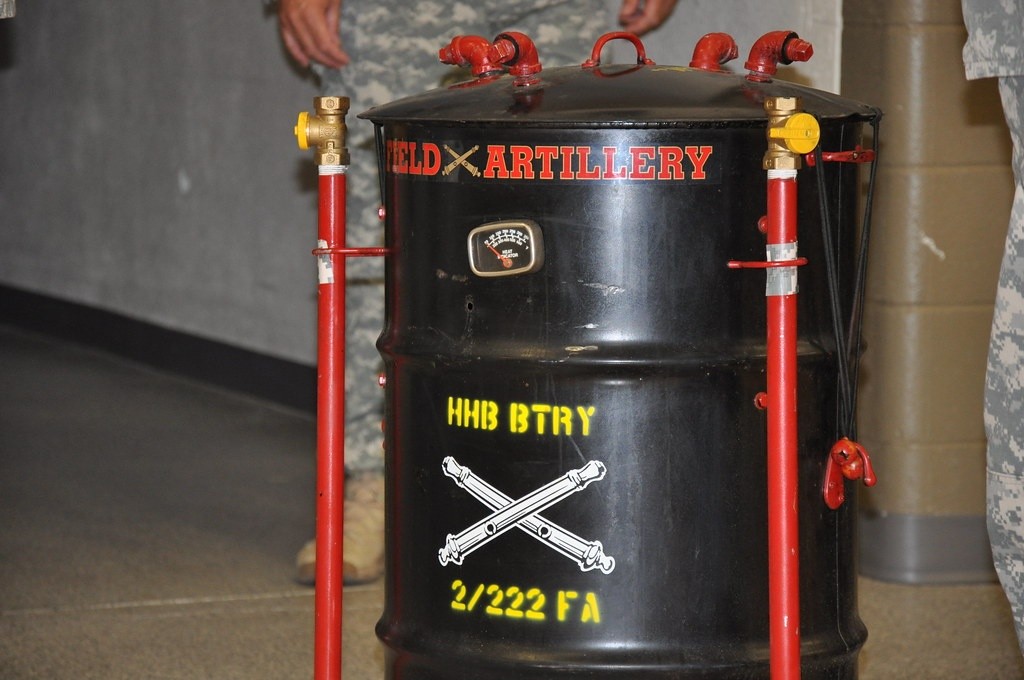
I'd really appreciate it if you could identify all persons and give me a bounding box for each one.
[276,1,677,592]
[962,1,1024,652]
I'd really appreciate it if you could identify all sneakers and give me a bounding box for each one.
[295,471,385,585]
